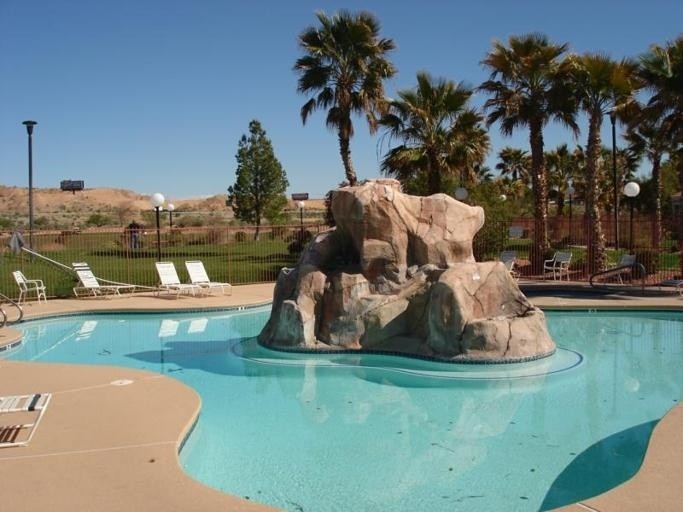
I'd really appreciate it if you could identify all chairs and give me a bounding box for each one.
[152,260,233,300]
[542,250,573,281]
[11,270,48,305]
[71,261,136,300]
[500,251,517,279]
[597,254,637,284]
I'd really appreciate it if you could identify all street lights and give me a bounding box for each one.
[607,112,619,252]
[167,205,174,226]
[299,201,304,232]
[455,188,468,203]
[499,195,507,261]
[21,121,37,261]
[151,194,164,262]
[566,187,575,240]
[623,182,641,253]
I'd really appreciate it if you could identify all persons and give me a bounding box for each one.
[127,219,140,248]
